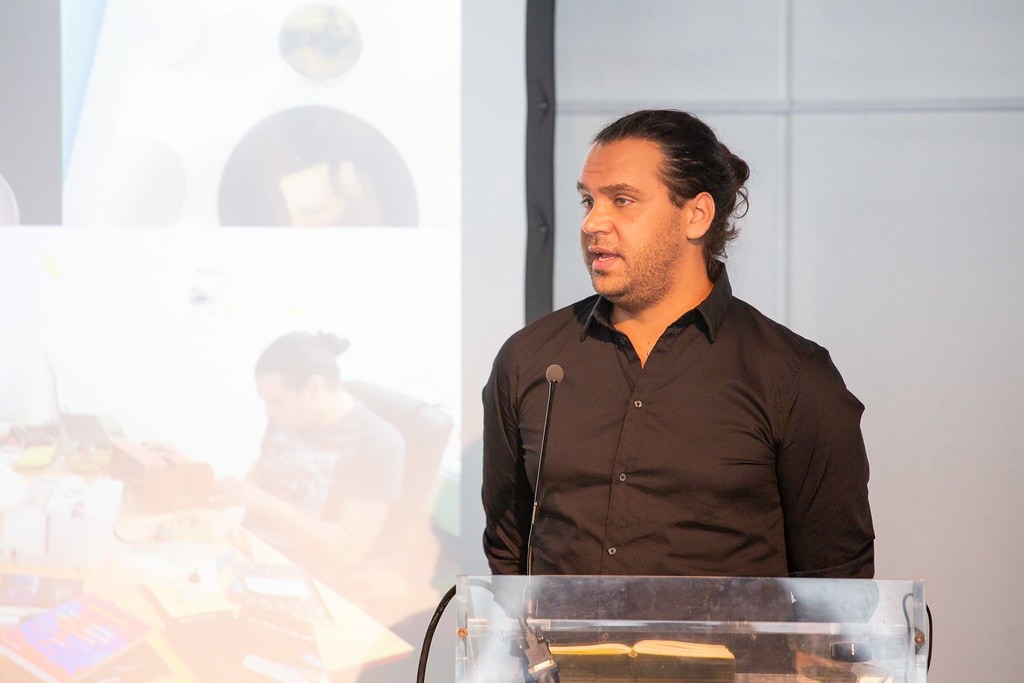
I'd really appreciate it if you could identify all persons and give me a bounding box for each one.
[481,109,874,675]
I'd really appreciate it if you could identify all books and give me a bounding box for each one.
[546,638,735,670]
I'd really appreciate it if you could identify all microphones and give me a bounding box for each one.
[529,363,564,576]
